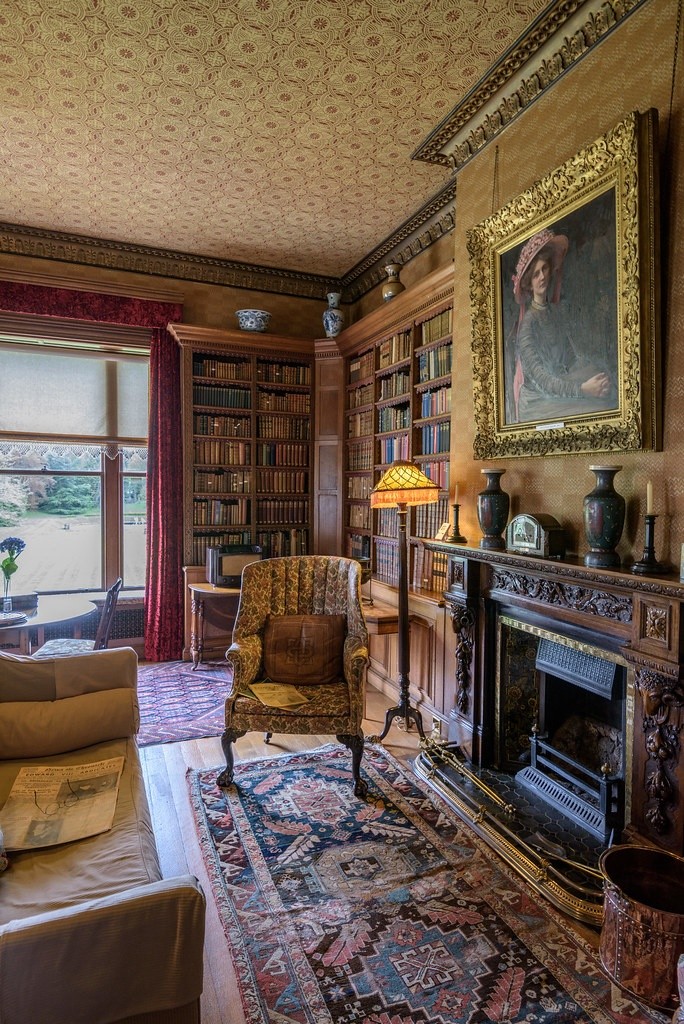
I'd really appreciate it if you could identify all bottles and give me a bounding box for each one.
[322,293,345,338]
[382,263,405,302]
[477,468,510,551]
[582,464,626,567]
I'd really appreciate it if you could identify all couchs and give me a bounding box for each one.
[0,649,206,1024]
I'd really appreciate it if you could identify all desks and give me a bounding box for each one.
[0,598,98,655]
[359,593,412,636]
[187,583,241,671]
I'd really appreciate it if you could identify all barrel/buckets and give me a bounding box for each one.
[598,842,684,1012]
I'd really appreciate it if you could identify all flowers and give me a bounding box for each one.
[0,536,26,596]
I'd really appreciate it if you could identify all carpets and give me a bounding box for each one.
[135,655,235,749]
[185,737,671,1024]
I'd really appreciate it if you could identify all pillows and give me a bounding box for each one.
[258,612,346,683]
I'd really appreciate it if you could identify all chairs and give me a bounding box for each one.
[215,553,368,797]
[31,578,122,657]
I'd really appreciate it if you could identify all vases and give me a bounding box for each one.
[477,468,510,550]
[322,292,345,338]
[381,263,406,304]
[3,578,13,613]
[583,465,626,568]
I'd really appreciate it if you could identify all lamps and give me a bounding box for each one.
[370,460,442,748]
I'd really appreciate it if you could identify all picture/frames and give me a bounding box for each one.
[465,109,663,461]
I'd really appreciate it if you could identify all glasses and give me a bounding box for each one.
[34,779,79,816]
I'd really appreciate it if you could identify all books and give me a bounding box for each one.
[345,310,451,596]
[190,357,311,566]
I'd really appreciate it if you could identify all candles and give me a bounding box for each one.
[647,481,653,513]
[455,485,459,503]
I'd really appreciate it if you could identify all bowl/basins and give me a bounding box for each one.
[234,309,273,333]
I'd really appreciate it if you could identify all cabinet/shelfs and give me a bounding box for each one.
[167,260,457,636]
